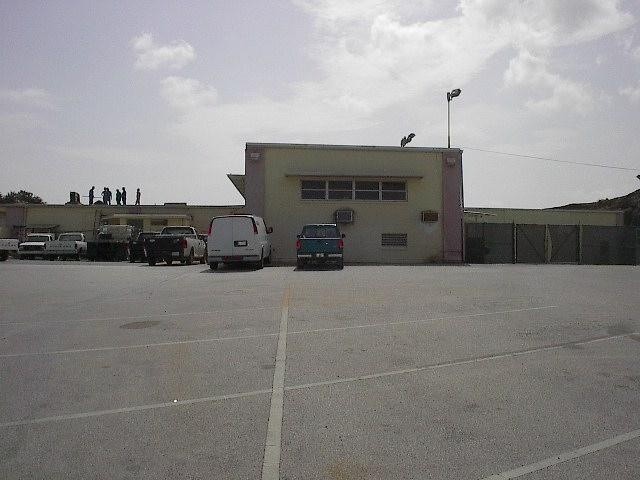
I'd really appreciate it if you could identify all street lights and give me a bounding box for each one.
[447,89,462,148]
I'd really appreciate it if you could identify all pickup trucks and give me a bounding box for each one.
[0,233,87,262]
[129,226,206,266]
[295,223,346,269]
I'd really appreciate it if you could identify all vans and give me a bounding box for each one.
[206,214,273,270]
[86,225,134,261]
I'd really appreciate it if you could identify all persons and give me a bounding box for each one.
[134,188,141,207]
[106,188,113,205]
[88,185,95,205]
[116,188,122,206]
[121,186,127,205]
[101,187,110,204]
